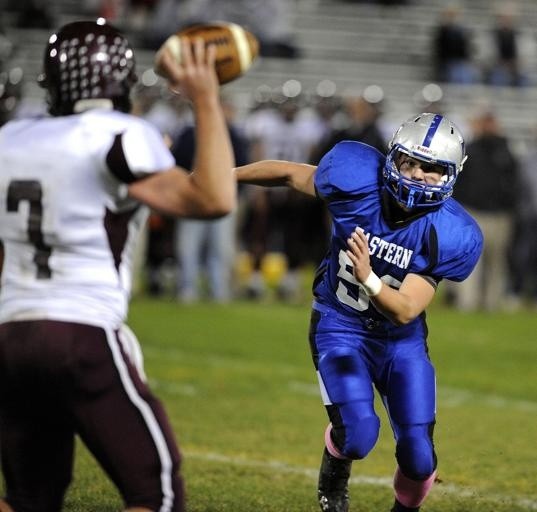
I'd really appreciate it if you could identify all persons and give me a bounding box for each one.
[1,0,301,61]
[1,20,237,510]
[232,110,487,512]
[484,16,526,86]
[1,82,519,314]
[430,9,477,83]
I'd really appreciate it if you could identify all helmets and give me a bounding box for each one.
[37,20,140,117]
[382,112,468,208]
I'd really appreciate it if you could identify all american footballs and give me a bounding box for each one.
[155,21,260,85]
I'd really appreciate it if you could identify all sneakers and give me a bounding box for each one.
[317,445,352,511]
